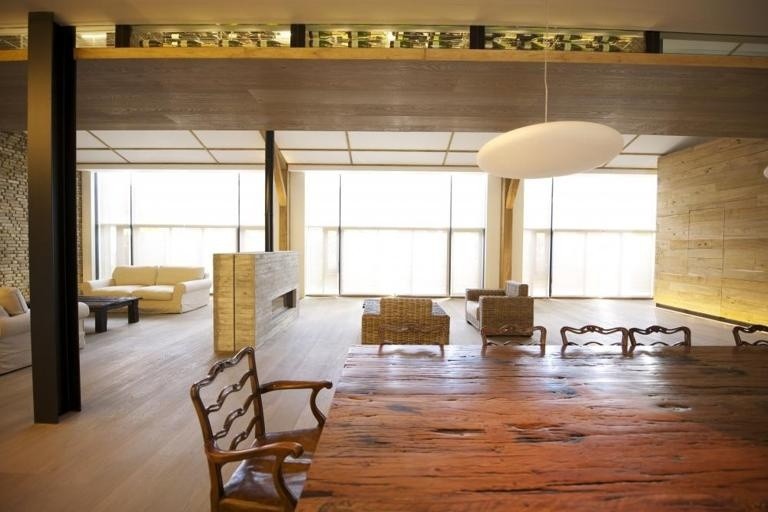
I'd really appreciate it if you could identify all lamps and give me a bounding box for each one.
[476,1,625,181]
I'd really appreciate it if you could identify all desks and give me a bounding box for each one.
[296,343,768,511]
[25,296,144,334]
[81,264,212,314]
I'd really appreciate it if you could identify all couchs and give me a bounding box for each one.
[0,285,90,376]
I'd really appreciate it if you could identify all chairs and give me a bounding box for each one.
[629,325,692,348]
[480,323,547,347]
[376,323,450,346]
[191,347,334,510]
[560,324,629,347]
[732,323,767,346]
[361,280,534,345]
